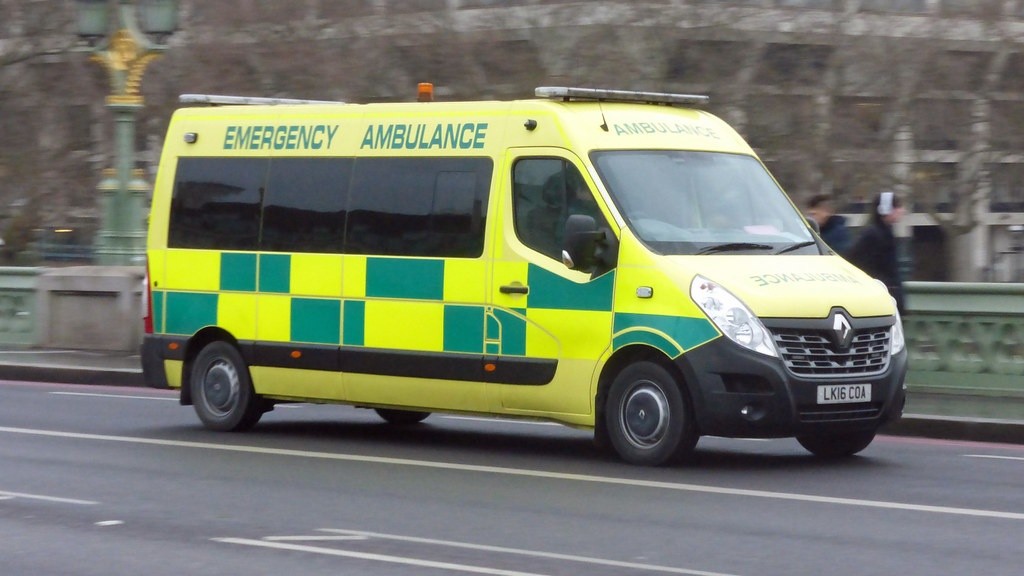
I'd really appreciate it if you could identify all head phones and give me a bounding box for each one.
[877,192,894,215]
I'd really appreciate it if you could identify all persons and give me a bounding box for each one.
[552,159,643,265]
[848,192,906,322]
[804,194,849,261]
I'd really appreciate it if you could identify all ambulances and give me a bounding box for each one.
[137,86,912,471]
